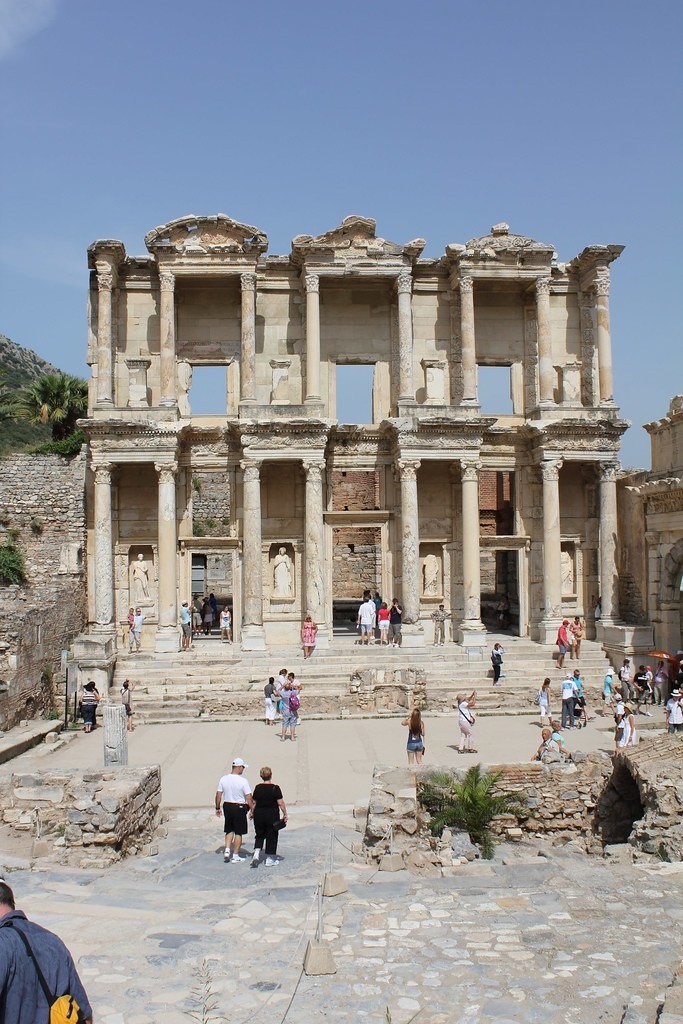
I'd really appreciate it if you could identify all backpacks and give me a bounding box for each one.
[289,692,300,711]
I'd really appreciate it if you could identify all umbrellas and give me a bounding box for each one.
[647,650,675,670]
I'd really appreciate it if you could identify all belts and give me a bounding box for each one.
[224,802,240,805]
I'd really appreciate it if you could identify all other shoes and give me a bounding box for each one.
[457,748,477,754]
[556,664,568,669]
[492,683,501,686]
[180,632,233,653]
[636,701,665,718]
[265,718,300,742]
[361,641,445,648]
[600,713,608,717]
[540,720,589,730]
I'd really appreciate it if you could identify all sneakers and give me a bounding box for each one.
[224,852,230,861]
[232,857,245,864]
[250,857,258,868]
[265,859,279,866]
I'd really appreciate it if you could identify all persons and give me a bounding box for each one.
[356,591,403,648]
[219,605,233,645]
[215,757,289,868]
[555,616,584,669]
[456,690,478,754]
[591,595,601,620]
[302,615,318,659]
[180,592,218,652]
[264,668,303,742]
[0,874,92,1024]
[431,605,448,647]
[492,643,506,687]
[126,608,157,653]
[401,708,425,765]
[78,681,101,733]
[120,679,136,732]
[532,646,683,762]
[273,547,291,598]
[495,594,510,630]
[133,554,153,603]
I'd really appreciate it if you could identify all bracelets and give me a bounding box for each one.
[216,808,220,810]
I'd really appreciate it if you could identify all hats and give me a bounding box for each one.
[563,620,571,625]
[670,689,682,697]
[439,603,444,607]
[605,658,651,714]
[455,692,466,703]
[232,758,248,767]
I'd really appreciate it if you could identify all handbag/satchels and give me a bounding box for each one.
[470,716,475,725]
[491,655,503,665]
[421,746,426,755]
[273,819,286,831]
[49,994,81,1024]
[271,685,283,702]
